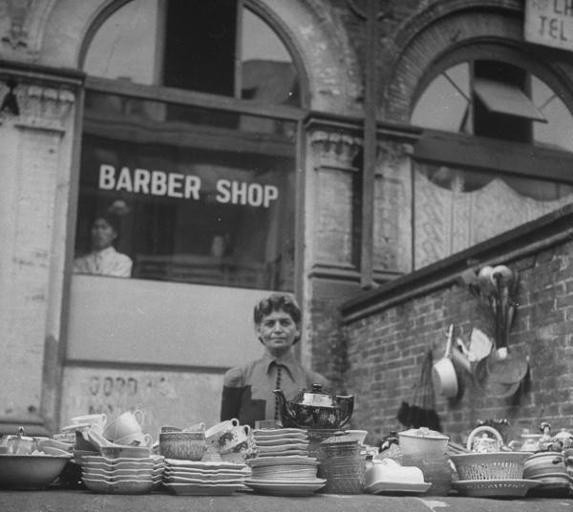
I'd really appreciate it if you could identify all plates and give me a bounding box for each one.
[365,479,431,494]
[242,427,327,496]
[78,456,249,498]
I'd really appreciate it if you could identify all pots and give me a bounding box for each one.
[431,324,459,398]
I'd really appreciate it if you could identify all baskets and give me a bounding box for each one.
[451,446,530,479]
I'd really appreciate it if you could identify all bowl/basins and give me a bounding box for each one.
[522,452,572,495]
[0,452,71,490]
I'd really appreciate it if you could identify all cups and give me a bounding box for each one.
[53,406,251,463]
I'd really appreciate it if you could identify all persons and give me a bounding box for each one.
[220,291,331,428]
[68,205,133,279]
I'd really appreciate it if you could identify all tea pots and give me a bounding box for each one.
[272,383,354,433]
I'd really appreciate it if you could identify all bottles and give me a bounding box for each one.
[321,431,367,494]
[394,426,450,457]
